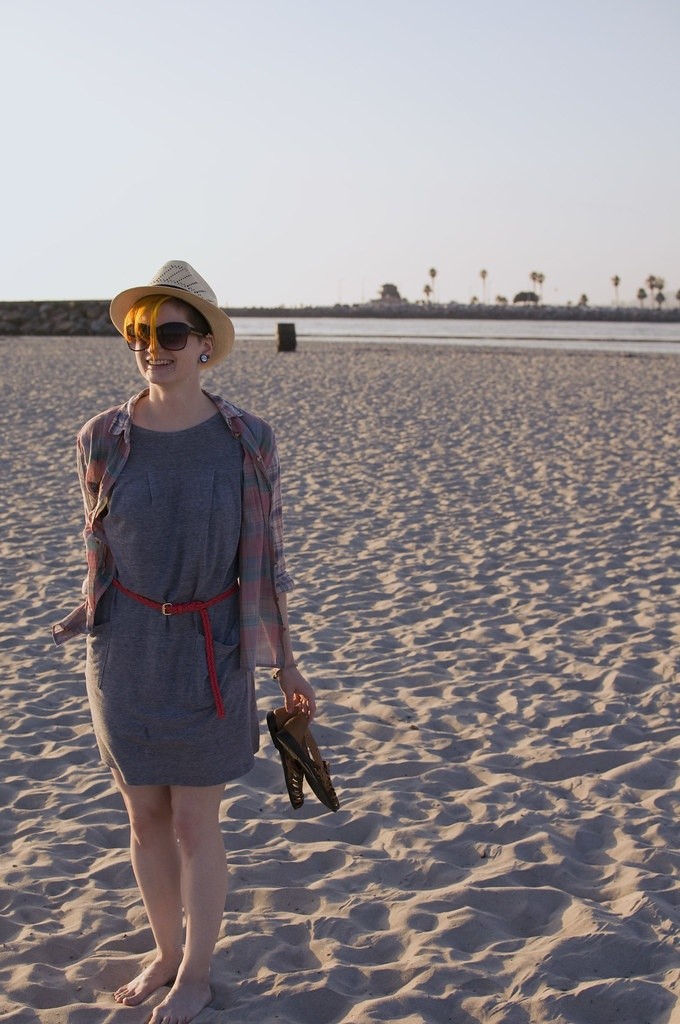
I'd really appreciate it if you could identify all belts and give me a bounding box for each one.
[110,578,238,719]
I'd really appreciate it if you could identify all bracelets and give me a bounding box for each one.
[273,664,298,679]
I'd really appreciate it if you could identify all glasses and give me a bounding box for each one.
[125,321,205,351]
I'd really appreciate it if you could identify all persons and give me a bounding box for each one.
[53,260,316,1024]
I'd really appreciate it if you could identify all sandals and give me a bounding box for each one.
[277,710,341,813]
[267,702,305,810]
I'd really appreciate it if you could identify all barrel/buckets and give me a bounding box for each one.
[278,324,296,352]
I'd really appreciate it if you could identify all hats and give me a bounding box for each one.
[109,260,235,371]
